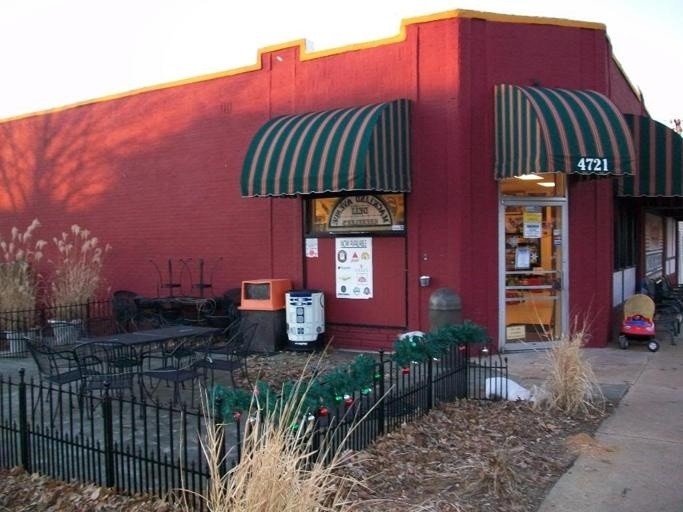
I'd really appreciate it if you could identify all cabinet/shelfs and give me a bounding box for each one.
[506,213,540,266]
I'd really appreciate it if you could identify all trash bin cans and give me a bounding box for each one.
[429,288,462,333]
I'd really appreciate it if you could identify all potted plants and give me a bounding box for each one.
[0,219,46,352]
[44,224,111,346]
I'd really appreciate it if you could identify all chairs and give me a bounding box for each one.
[14,287,257,427]
[642,274,682,345]
